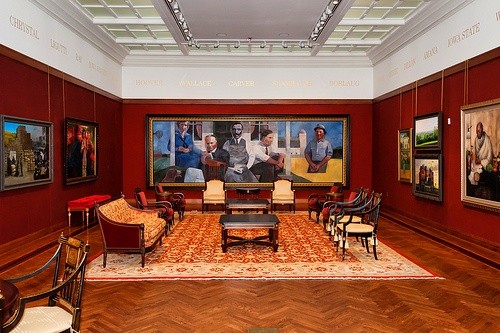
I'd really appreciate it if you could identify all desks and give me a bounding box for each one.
[0,278,19,322]
[68,194,110,226]
[237,188,260,212]
[219,214,280,253]
[226,199,269,214]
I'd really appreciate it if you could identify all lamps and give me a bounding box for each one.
[214,39,289,48]
[299,0,340,48]
[167,0,201,48]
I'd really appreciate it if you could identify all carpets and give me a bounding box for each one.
[56,211,447,280]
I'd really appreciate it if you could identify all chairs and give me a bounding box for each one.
[307,182,382,260]
[270,178,296,215]
[95,183,185,268]
[0,234,89,333]
[201,179,226,214]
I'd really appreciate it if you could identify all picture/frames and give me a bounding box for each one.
[460,98,500,213]
[145,112,351,190]
[398,112,443,202]
[0,115,55,192]
[64,117,99,187]
